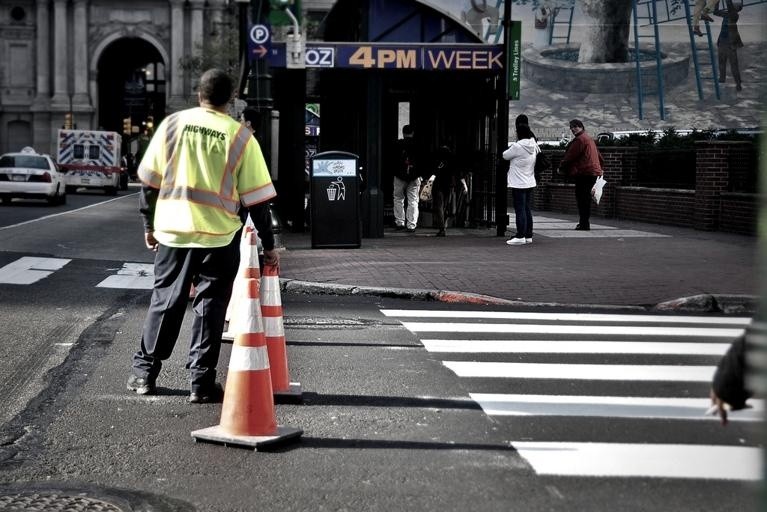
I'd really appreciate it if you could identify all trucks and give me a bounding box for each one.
[56,128,127,197]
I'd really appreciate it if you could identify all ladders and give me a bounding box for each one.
[639,0,767,28]
[549,0,575,44]
[633,0,664,121]
[485,1,506,46]
[682,0,719,101]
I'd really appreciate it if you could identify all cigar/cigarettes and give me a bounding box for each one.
[705,406,720,415]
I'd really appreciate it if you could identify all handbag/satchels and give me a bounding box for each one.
[535,152,551,173]
[420,175,436,200]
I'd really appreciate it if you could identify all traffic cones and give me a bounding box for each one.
[190,275,306,453]
[220,222,262,343]
[236,247,303,396]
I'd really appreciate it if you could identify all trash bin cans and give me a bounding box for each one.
[309,150,362,250]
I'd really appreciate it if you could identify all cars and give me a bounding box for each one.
[1,146,69,207]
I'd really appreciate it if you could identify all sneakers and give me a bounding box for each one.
[188,382,222,404]
[127,375,155,394]
[507,237,532,245]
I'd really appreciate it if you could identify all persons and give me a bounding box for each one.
[392,126,427,232]
[713,1,744,93]
[240,109,262,142]
[502,114,604,246]
[692,1,720,36]
[423,141,468,236]
[127,69,281,403]
[709,305,764,427]
